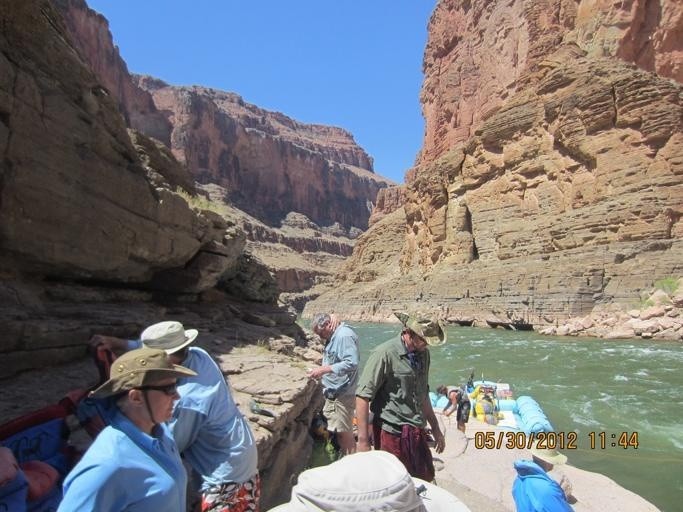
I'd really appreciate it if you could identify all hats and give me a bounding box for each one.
[265,450,472,512]
[394,311,447,347]
[436,384,445,395]
[140,320,199,354]
[87,347,198,400]
[527,437,568,465]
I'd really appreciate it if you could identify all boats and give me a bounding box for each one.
[436,380,555,437]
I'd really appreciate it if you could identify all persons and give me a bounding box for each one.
[86,318,261,511]
[304,310,360,458]
[352,306,446,483]
[511,436,575,512]
[57,347,197,511]
[435,382,471,433]
